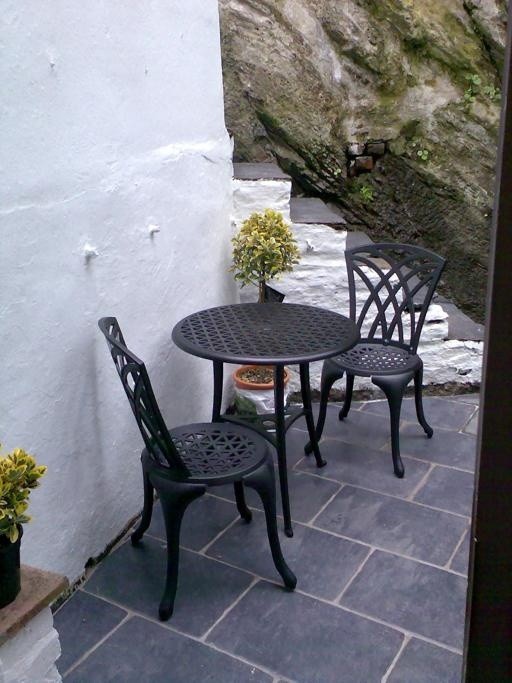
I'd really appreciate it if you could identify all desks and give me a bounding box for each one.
[168,297,366,543]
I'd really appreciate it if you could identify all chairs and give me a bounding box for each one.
[294,239,450,481]
[96,314,305,626]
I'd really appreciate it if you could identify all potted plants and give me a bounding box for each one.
[0,440,49,609]
[227,205,303,430]
